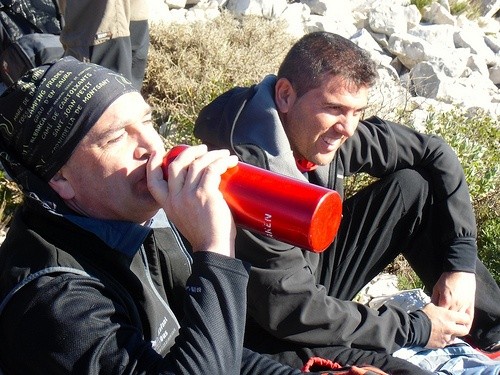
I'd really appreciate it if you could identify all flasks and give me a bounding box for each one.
[162,144,343,252]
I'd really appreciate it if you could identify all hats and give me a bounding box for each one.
[1,56,140,190]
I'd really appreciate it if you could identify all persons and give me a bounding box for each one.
[0,56,314,375]
[0,0,151,98]
[189,31,500,375]
